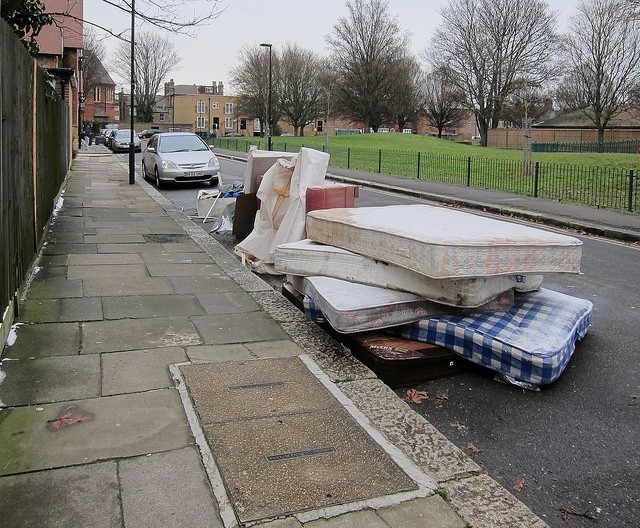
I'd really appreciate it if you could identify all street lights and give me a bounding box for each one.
[260,44,272,150]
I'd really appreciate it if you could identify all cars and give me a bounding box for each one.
[98,129,118,149]
[142,132,221,189]
[111,129,141,153]
[138,129,161,139]
[226,133,241,137]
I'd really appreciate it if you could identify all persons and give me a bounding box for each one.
[84,123,93,145]
